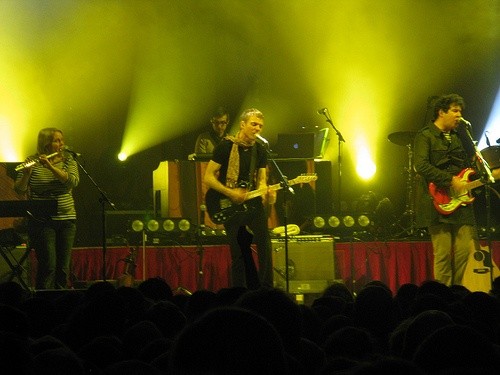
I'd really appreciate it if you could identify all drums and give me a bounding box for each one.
[468,185,500,237]
[478,146,500,185]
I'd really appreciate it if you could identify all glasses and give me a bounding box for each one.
[213,117,229,124]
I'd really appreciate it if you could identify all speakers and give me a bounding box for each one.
[270,234,335,293]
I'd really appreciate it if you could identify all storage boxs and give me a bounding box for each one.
[105,210,154,244]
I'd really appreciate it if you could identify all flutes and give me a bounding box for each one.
[13,150,61,173]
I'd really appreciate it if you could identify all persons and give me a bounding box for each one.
[194,110,230,154]
[204,109,276,298]
[413,94,476,288]
[0,274,500,375]
[13,128,79,290]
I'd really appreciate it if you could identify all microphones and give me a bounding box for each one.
[485,134,490,145]
[254,133,268,144]
[318,109,325,114]
[62,145,81,157]
[458,117,472,127]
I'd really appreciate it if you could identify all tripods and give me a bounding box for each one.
[386,144,431,241]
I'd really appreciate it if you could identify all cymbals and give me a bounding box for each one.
[387,132,417,146]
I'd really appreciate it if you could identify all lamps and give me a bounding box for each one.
[311,212,373,240]
[128,217,194,244]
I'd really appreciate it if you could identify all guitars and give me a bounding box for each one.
[204,172,318,224]
[459,239,500,293]
[429,168,500,216]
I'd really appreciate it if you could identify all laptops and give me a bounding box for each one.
[271,132,315,158]
